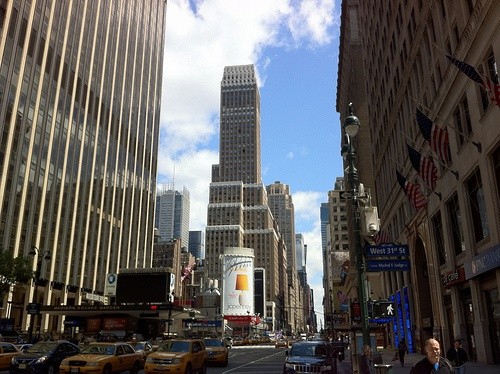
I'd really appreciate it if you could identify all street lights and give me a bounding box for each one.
[340,102,376,374]
[27,245,53,344]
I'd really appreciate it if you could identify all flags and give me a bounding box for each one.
[406,146,438,192]
[369,229,391,244]
[394,171,428,211]
[444,56,500,109]
[415,107,451,160]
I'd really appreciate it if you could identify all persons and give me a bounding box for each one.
[410,338,456,374]
[445,339,468,374]
[397,338,410,367]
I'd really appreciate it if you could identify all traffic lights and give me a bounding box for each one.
[378,301,396,318]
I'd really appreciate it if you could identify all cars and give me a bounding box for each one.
[284,340,338,374]
[11,340,81,374]
[132,341,157,368]
[144,339,206,374]
[15,343,34,352]
[231,333,309,346]
[59,342,142,374]
[0,342,21,371]
[203,338,230,366]
[274,339,289,349]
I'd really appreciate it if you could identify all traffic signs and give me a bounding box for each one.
[338,190,369,200]
